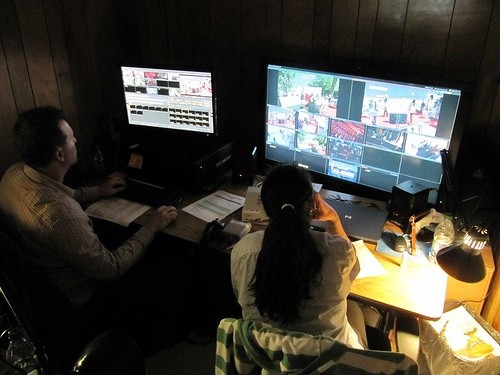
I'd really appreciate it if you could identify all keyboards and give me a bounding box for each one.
[115,177,183,209]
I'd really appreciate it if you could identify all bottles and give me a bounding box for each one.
[6,328,38,375]
[94,144,108,184]
[429,212,455,264]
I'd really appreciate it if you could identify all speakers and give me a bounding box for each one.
[389,180,429,228]
[232,143,257,186]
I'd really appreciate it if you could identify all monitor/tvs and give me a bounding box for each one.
[265,64,477,212]
[121,66,218,137]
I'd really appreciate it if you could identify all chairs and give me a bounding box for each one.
[214,317,418,375]
[0,209,149,375]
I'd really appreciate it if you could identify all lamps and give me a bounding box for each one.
[381,190,489,283]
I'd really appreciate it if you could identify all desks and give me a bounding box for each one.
[132,174,449,337]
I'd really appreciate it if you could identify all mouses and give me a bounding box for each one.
[392,236,407,252]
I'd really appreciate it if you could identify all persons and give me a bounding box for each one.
[368,93,434,126]
[230,165,386,353]
[0,105,178,360]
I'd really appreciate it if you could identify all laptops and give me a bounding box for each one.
[312,199,389,243]
